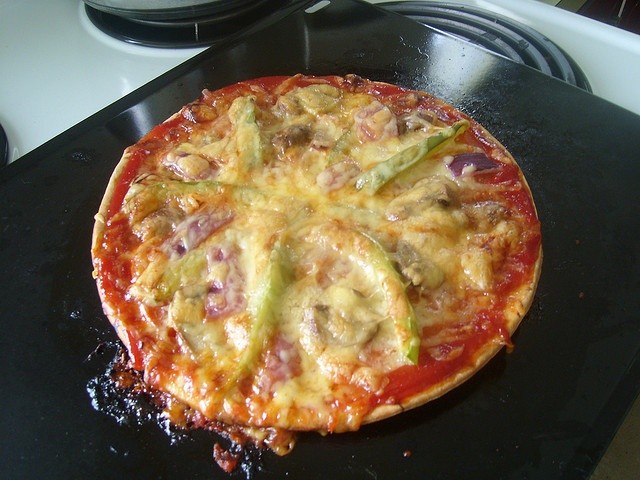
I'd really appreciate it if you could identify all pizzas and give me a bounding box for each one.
[90,74,543,430]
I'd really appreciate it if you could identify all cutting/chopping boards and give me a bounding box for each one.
[1,0,640,480]
